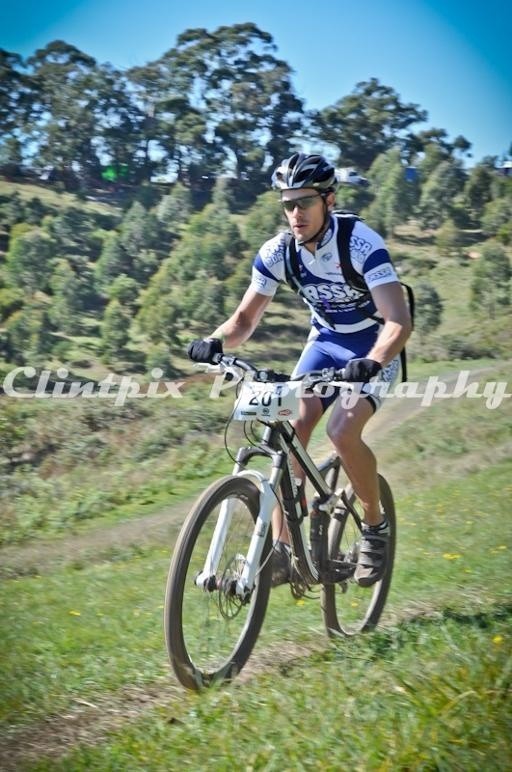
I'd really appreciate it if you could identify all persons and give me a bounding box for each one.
[185,153,415,587]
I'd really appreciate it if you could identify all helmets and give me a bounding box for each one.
[270,152,337,192]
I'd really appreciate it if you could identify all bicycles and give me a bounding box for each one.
[164,352,397,692]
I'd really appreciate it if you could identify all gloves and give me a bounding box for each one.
[187,337,223,365]
[344,358,383,383]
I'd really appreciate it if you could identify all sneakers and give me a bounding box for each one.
[354,514,392,588]
[269,541,295,586]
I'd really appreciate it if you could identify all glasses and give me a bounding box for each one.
[279,192,327,211]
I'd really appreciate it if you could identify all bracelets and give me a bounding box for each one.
[208,330,227,348]
